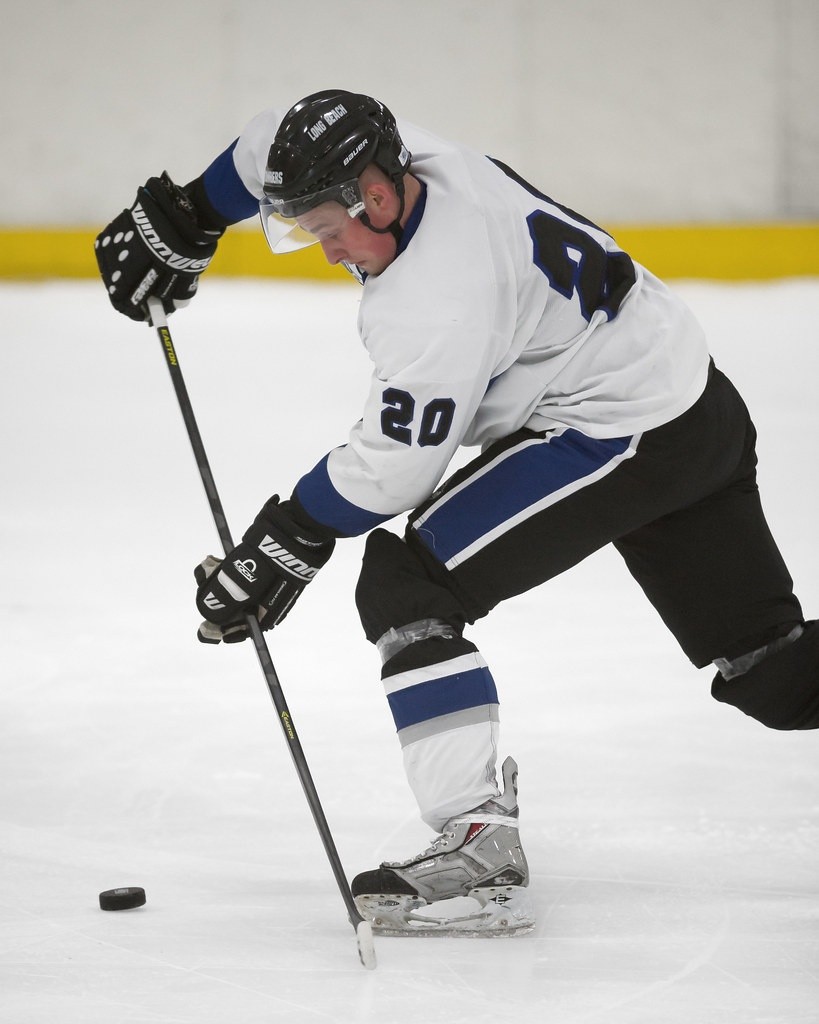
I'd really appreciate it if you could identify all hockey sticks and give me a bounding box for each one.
[148,298,387,975]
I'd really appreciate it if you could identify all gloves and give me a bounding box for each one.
[194,493,340,644]
[94,169,228,327]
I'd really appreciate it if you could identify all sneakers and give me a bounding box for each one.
[350,755,538,938]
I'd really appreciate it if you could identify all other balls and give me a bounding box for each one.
[90,884,154,915]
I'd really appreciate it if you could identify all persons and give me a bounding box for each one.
[92,89,819,934]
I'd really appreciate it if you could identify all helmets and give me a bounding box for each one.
[263,89,413,217]
